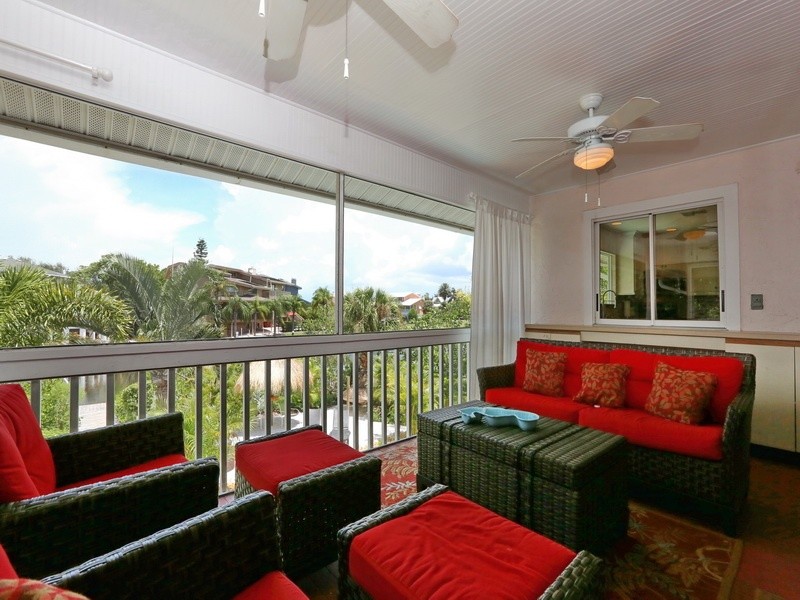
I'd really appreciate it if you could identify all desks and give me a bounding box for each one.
[415,399,630,554]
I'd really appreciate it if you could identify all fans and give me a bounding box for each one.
[252,0,459,60]
[511,93,704,181]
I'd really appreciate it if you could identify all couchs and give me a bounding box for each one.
[477,338,757,520]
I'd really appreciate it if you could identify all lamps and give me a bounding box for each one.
[682,228,705,240]
[574,139,615,205]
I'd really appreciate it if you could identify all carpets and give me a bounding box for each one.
[381,438,742,600]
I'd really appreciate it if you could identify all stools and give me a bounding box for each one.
[235,424,382,578]
[336,483,603,600]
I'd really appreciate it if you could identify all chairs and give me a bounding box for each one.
[0,384,219,579]
[0,490,310,600]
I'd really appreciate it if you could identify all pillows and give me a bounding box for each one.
[572,361,632,408]
[522,348,567,396]
[643,361,718,423]
